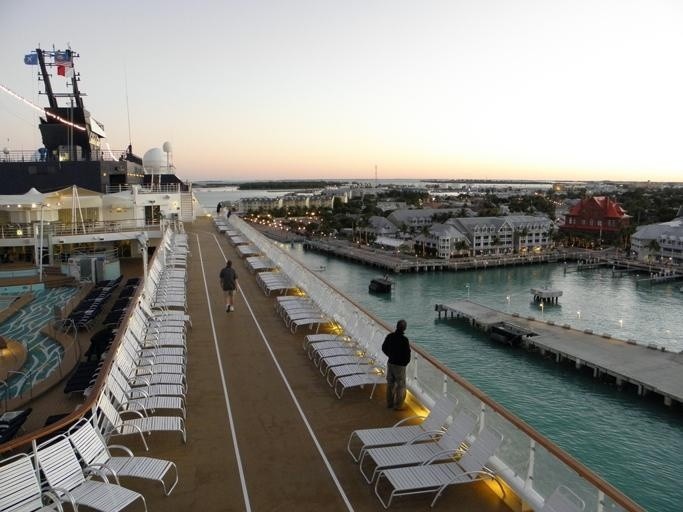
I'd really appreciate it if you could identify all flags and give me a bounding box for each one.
[23,52,73,77]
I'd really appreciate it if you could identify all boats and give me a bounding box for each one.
[368,273,392,293]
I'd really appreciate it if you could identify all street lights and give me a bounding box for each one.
[38,203,51,280]
[617,319,622,342]
[575,310,581,330]
[465,283,470,301]
[539,302,544,318]
[131,181,143,227]
[505,295,511,304]
[133,229,148,282]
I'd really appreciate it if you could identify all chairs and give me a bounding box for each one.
[67,416,179,497]
[540,484,585,511]
[208,209,272,257]
[340,390,507,511]
[1,217,194,452]
[35,434,149,511]
[243,254,405,401]
[0,452,65,512]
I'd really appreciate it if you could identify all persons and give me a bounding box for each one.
[219,260,239,313]
[382,319,411,410]
